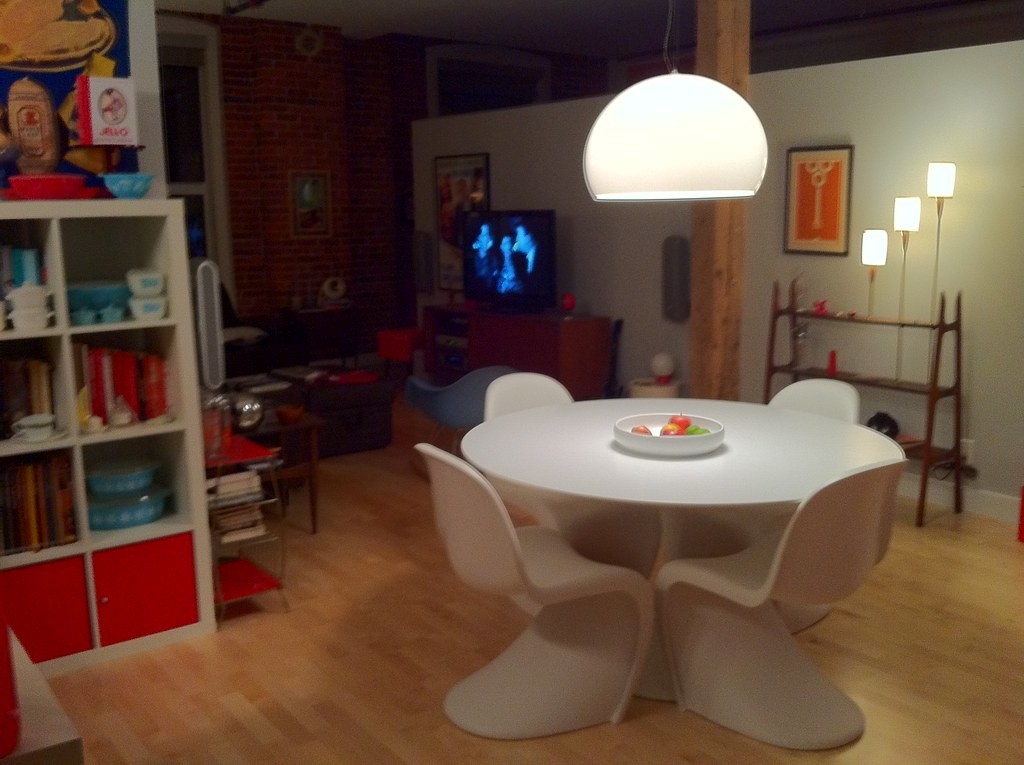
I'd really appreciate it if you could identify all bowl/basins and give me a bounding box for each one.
[8,174,87,198]
[98,173,154,197]
[126,268,167,320]
[87,455,172,529]
[5,280,54,331]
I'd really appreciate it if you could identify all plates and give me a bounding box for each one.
[613,412,724,456]
[9,432,69,444]
[0,0,118,73]
[0,187,100,198]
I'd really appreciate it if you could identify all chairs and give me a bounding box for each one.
[652,458,910,751]
[412,443,658,741]
[727,374,859,538]
[480,371,637,564]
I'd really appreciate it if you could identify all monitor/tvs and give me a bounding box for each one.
[461,208,558,314]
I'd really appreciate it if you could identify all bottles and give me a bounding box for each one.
[108,396,131,425]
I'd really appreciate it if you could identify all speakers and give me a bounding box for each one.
[662,236,690,322]
[411,233,434,295]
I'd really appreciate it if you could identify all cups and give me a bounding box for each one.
[11,413,53,440]
[81,416,101,432]
[277,400,303,424]
[71,304,123,325]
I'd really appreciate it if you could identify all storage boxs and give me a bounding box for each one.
[75,73,138,147]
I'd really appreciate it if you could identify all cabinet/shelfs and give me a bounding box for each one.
[419,299,616,396]
[0,197,218,678]
[760,278,964,529]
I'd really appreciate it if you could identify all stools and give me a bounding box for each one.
[292,369,391,457]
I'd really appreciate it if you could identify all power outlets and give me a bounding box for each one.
[960,439,975,466]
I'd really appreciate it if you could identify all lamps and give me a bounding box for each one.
[581,0,768,202]
[863,158,957,381]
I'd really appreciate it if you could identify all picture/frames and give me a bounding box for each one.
[435,151,488,293]
[288,169,335,242]
[780,143,856,256]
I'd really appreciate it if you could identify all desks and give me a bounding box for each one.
[460,395,907,704]
[233,396,327,535]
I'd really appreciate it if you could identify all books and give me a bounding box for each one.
[72,342,167,427]
[206,465,266,545]
[0,358,56,441]
[0,455,78,557]
